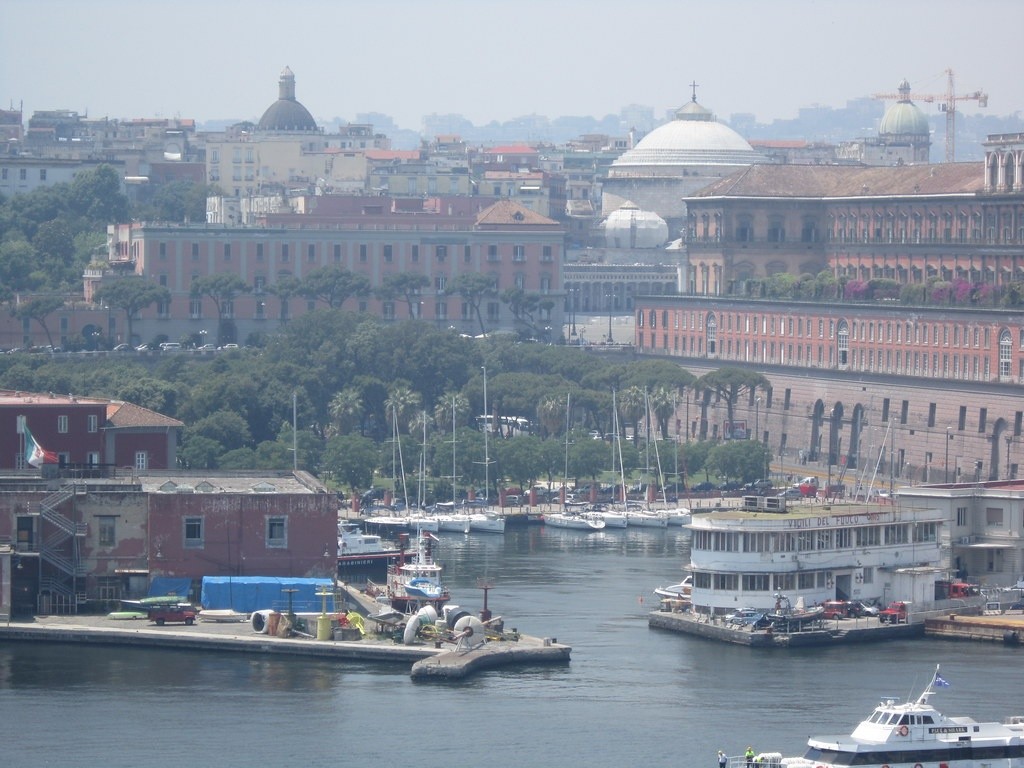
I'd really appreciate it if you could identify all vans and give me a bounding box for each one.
[724,608,755,622]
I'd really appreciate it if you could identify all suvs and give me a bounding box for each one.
[745,479,773,489]
[148,602,196,626]
[777,489,800,500]
[793,477,819,489]
[817,602,847,620]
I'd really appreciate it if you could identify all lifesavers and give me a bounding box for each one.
[914,764,923,768]
[900,726,909,736]
[882,765,889,768]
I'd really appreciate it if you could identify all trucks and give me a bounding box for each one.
[879,601,912,624]
[935,581,980,610]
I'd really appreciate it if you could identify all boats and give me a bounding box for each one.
[766,586,825,629]
[337,516,420,570]
[714,659,1024,767]
[729,611,757,626]
[655,585,691,602]
[691,481,714,491]
[716,480,741,490]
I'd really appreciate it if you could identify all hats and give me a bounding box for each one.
[747,747,751,751]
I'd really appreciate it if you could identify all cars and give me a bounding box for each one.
[859,601,880,616]
[845,601,860,617]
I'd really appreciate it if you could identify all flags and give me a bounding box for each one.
[23,423,60,470]
[934,674,951,689]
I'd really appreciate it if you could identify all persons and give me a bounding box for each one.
[753,757,765,768]
[717,751,728,768]
[745,747,755,768]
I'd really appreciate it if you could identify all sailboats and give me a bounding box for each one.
[366,364,696,531]
[386,453,450,617]
